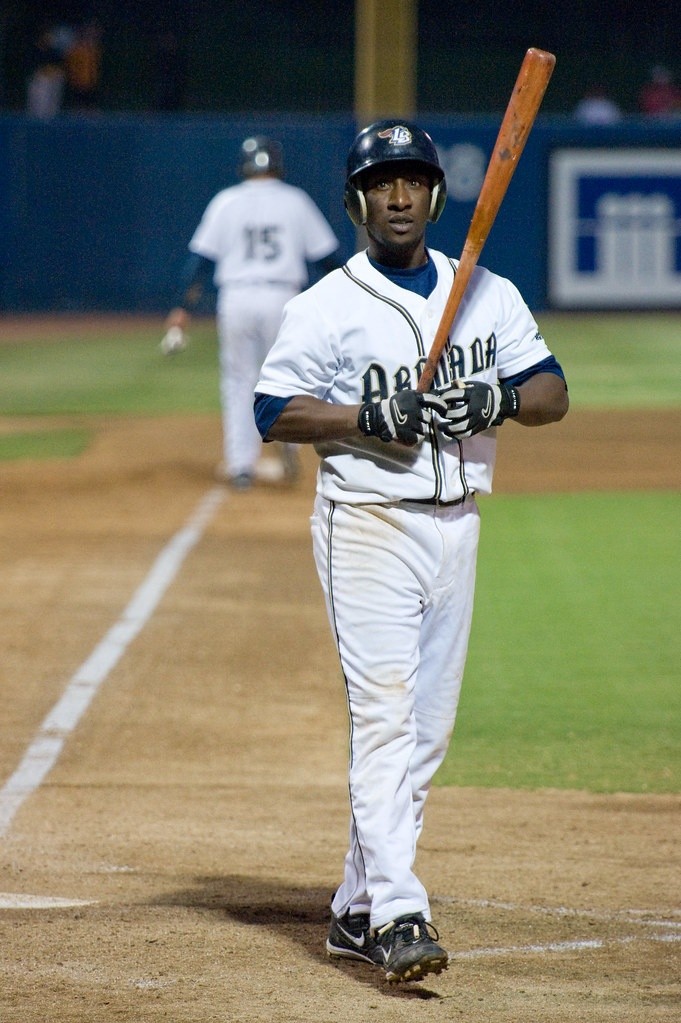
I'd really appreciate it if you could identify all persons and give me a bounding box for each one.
[166,130,343,493]
[573,78,619,118]
[27,26,94,112]
[638,62,681,114]
[252,116,569,983]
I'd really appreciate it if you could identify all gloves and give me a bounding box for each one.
[357,380,521,448]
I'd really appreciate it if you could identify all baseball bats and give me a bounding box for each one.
[412,45,559,377]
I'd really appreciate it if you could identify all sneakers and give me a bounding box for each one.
[327,914,380,966]
[372,913,449,984]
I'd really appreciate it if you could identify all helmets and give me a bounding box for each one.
[241,136,285,173]
[344,119,447,224]
